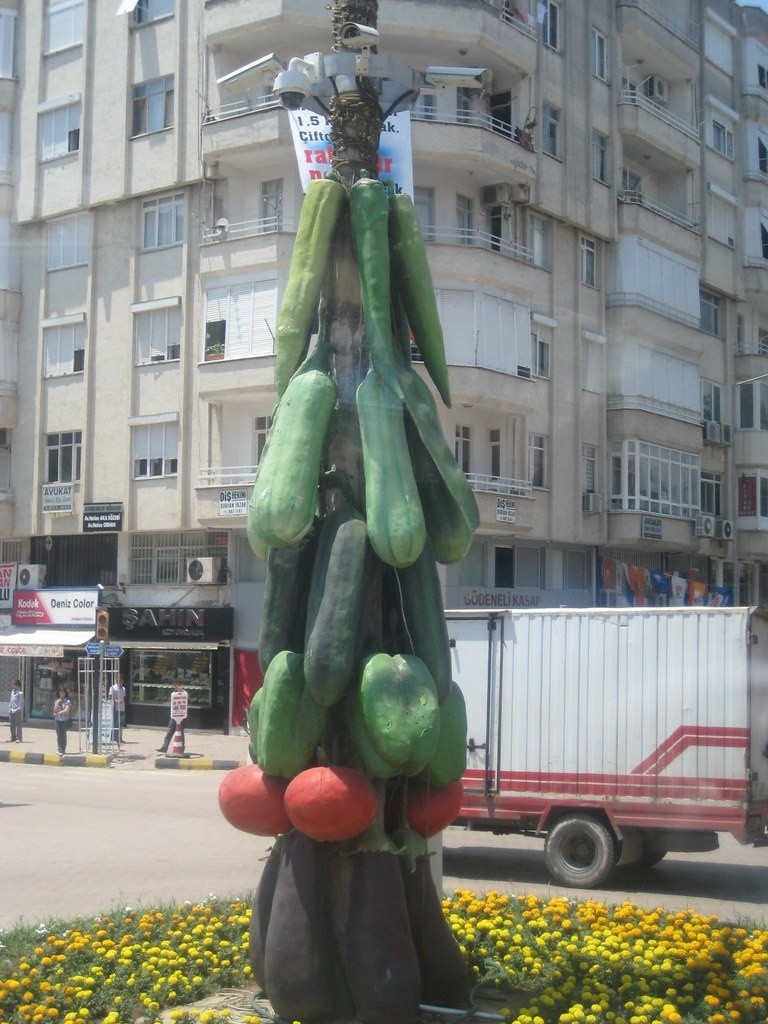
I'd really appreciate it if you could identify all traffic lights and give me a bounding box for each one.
[96,609,109,641]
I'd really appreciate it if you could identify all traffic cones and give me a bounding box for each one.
[169,722,188,759]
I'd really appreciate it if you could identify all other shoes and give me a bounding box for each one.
[57,749,64,756]
[155,748,167,753]
[5,738,22,743]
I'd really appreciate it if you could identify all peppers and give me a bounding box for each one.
[274,170,457,415]
[246,652,469,790]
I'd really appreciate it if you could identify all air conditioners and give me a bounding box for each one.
[702,420,720,443]
[720,422,732,445]
[480,181,511,208]
[695,515,714,538]
[186,556,222,584]
[17,563,48,590]
[714,518,735,541]
[647,74,668,103]
[581,492,602,513]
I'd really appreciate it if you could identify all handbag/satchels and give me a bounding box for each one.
[68,718,72,728]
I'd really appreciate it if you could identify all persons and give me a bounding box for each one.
[92,677,108,699]
[155,678,188,753]
[53,686,70,756]
[109,674,126,742]
[7,679,24,742]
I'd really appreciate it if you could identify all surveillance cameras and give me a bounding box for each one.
[272,70,311,112]
[337,21,380,60]
[96,583,105,594]
[417,65,493,97]
[118,572,126,587]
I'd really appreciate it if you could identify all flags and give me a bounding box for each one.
[602,557,733,606]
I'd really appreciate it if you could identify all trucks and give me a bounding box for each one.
[438,590,762,890]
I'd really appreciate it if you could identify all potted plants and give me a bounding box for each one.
[206,344,224,361]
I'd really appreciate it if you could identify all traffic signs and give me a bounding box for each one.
[85,643,101,655]
[104,645,124,657]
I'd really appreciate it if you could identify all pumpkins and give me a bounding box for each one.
[246,299,481,708]
[218,763,465,846]
